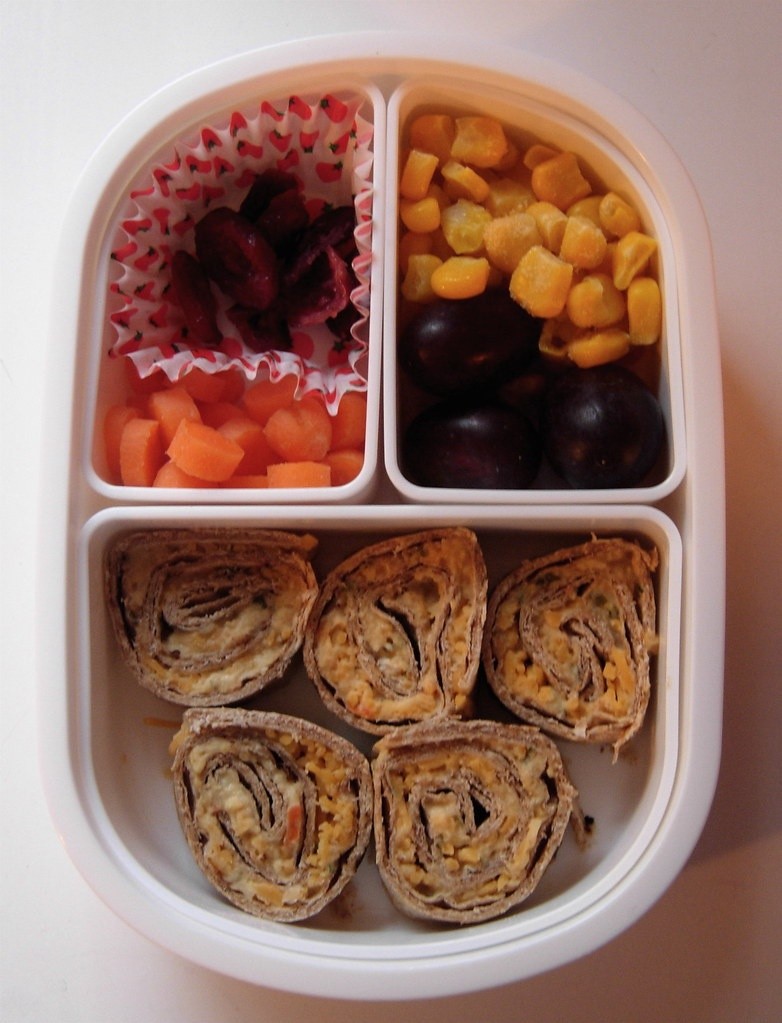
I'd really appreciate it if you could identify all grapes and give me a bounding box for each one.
[398,291,668,495]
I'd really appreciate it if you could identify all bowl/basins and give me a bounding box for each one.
[40,26,724,1000]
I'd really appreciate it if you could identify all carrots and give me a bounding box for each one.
[103,359,365,489]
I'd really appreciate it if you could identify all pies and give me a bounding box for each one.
[100,525,661,928]
